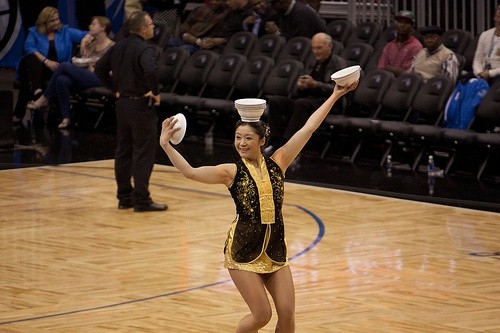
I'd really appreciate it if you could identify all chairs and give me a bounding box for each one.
[13,19,500,195]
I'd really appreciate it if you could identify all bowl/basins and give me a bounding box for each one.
[234,98,267,122]
[330,65,361,86]
[169,113,187,144]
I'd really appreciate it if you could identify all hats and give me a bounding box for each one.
[420,26,444,36]
[394,10,416,26]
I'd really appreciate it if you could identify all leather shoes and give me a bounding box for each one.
[133,202,167,212]
[118,198,136,209]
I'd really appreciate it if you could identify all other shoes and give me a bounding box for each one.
[286,156,301,173]
[27,100,49,111]
[58,123,69,129]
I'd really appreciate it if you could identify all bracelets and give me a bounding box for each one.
[43,58,48,63]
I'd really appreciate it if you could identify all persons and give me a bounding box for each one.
[160,80,361,333]
[7,7,96,131]
[27,16,115,128]
[407,24,459,86]
[284,33,348,166]
[107,0,328,53]
[470,5,500,83]
[376,10,424,77]
[94,11,167,212]
